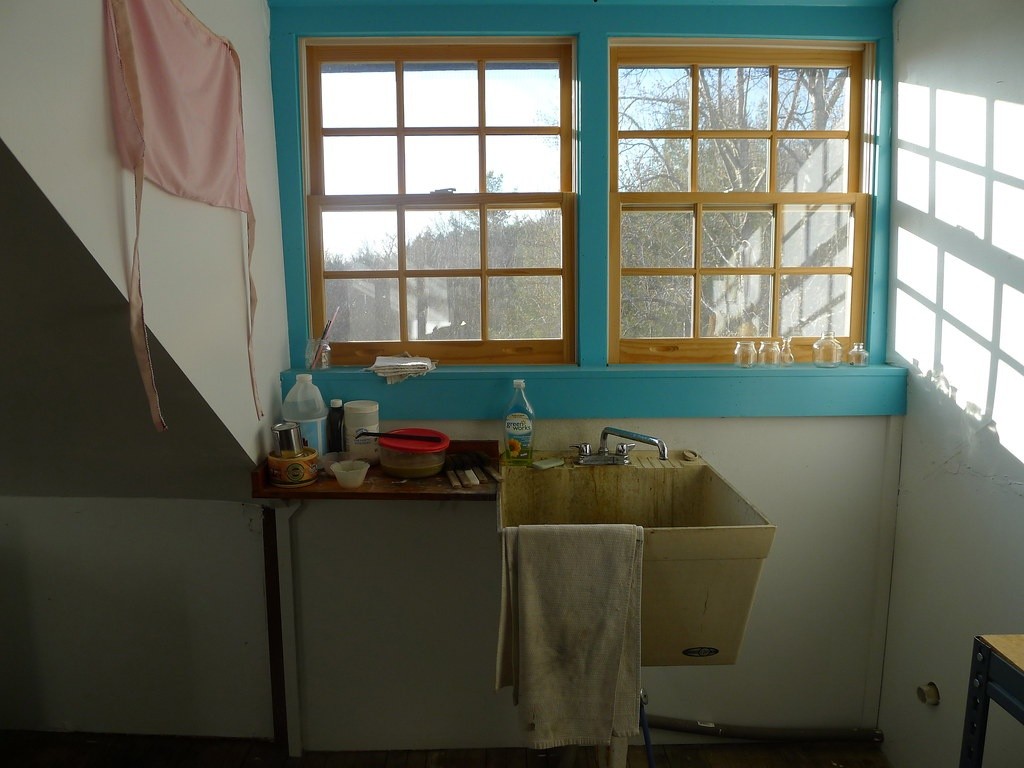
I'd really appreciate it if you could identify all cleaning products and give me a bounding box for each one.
[503,378,537,467]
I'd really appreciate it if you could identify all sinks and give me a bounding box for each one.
[498,462,777,670]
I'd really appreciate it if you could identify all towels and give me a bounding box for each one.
[494,524,642,751]
[363,350,440,385]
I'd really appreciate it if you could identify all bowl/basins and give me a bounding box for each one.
[377,428,449,478]
[329,460,370,489]
[320,452,353,480]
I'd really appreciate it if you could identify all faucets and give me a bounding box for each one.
[568,426,669,465]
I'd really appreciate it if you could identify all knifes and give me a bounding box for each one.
[444,450,503,488]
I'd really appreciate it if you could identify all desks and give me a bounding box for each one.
[959,634,1024,768]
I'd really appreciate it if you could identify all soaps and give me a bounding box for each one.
[531,456,565,470]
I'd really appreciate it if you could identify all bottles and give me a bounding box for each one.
[847,342,869,367]
[328,399,345,452]
[306,339,331,371]
[344,400,380,465]
[782,335,795,367]
[733,341,757,368]
[282,374,328,469]
[758,341,781,368]
[812,331,842,368]
[503,379,536,467]
